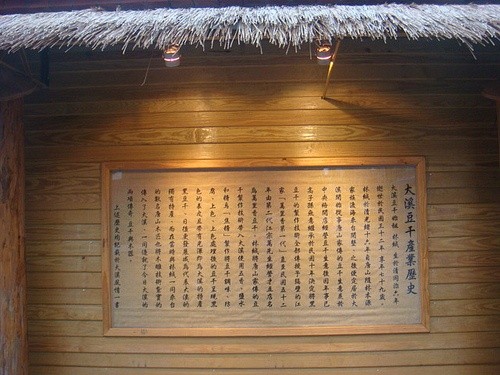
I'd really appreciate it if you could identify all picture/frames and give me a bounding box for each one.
[101,156,429,337]
